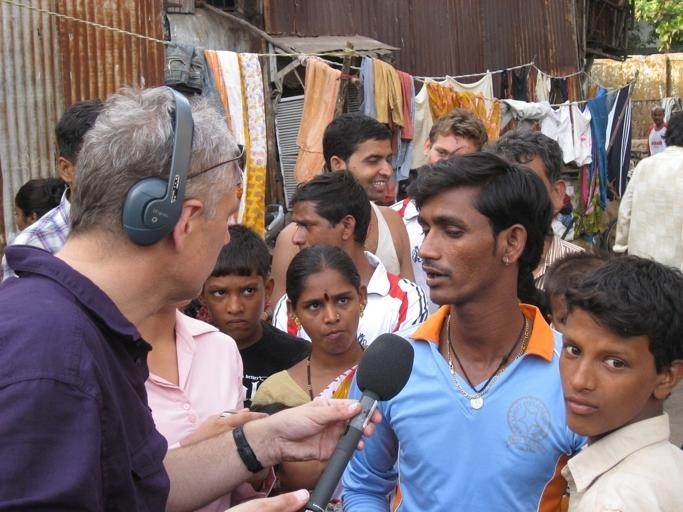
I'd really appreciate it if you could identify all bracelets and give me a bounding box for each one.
[232,423,265,474]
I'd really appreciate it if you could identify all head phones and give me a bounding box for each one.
[119,84,194,246]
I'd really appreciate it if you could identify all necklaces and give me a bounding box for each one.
[307,342,366,400]
[446,315,529,400]
[448,312,526,410]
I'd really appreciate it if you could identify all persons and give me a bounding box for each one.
[11,178,70,233]
[248,242,398,512]
[274,105,683,350]
[0,86,382,510]
[1,99,111,283]
[136,299,277,511]
[197,224,313,408]
[559,258,683,512]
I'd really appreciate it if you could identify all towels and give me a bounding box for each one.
[292,57,341,188]
[204,49,268,242]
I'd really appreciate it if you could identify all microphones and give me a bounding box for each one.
[306,330,416,512]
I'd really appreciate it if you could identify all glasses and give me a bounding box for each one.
[188,145,247,180]
